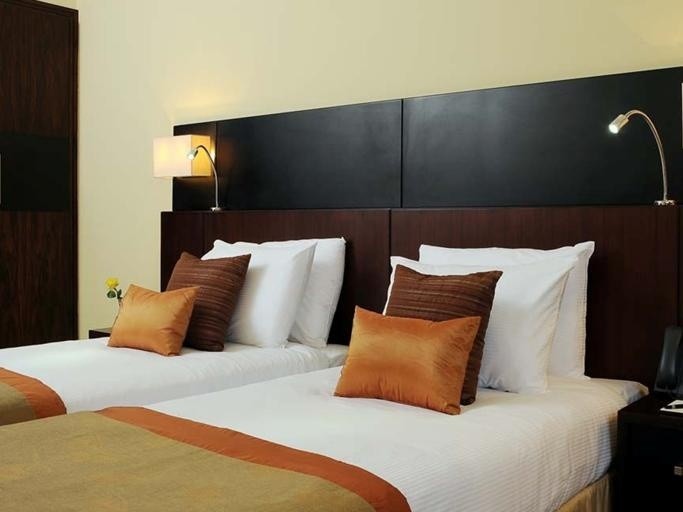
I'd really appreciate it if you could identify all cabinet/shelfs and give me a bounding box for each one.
[0,0,80,350]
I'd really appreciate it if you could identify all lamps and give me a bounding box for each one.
[605,110,677,208]
[150,130,214,180]
[184,142,223,214]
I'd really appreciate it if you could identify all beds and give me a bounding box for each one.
[0,202,683,511]
[0,208,393,425]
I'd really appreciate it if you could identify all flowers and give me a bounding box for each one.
[104,276,123,299]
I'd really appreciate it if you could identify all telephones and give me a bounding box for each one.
[653,325,683,400]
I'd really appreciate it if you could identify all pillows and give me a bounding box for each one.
[386,254,577,396]
[165,249,252,353]
[203,238,319,351]
[417,240,598,380]
[103,283,199,356]
[232,237,346,348]
[331,305,483,416]
[382,265,503,405]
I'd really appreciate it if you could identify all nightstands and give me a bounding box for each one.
[615,385,682,511]
[87,325,114,338]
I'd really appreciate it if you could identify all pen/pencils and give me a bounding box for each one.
[663,404,683,409]
